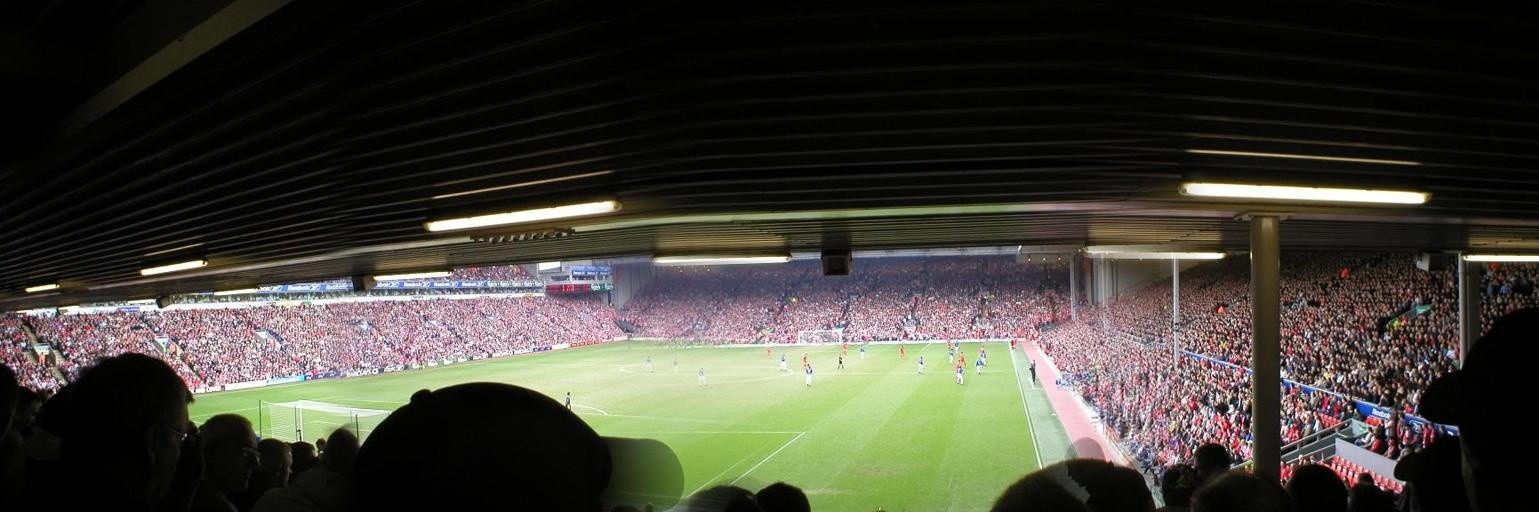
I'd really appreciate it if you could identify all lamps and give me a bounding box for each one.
[1462,244,1539,266]
[138,256,211,275]
[370,270,455,284]
[422,200,622,236]
[646,253,794,267]
[1170,179,1437,210]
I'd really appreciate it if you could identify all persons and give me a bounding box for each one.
[787,255,1539,511]
[2,255,813,511]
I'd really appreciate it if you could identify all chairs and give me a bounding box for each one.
[1037,244,1464,500]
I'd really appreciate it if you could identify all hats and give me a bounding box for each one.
[1394,433,1461,482]
[343,382,682,510]
[1418,307,1539,426]
